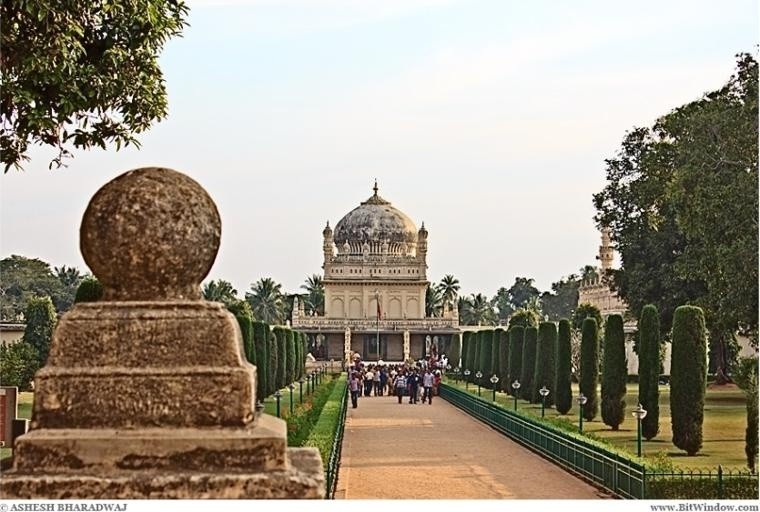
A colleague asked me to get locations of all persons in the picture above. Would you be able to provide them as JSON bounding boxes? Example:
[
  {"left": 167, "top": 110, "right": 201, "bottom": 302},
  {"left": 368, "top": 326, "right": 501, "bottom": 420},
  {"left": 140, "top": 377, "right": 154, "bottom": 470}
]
[{"left": 344, "top": 352, "right": 452, "bottom": 411}]
